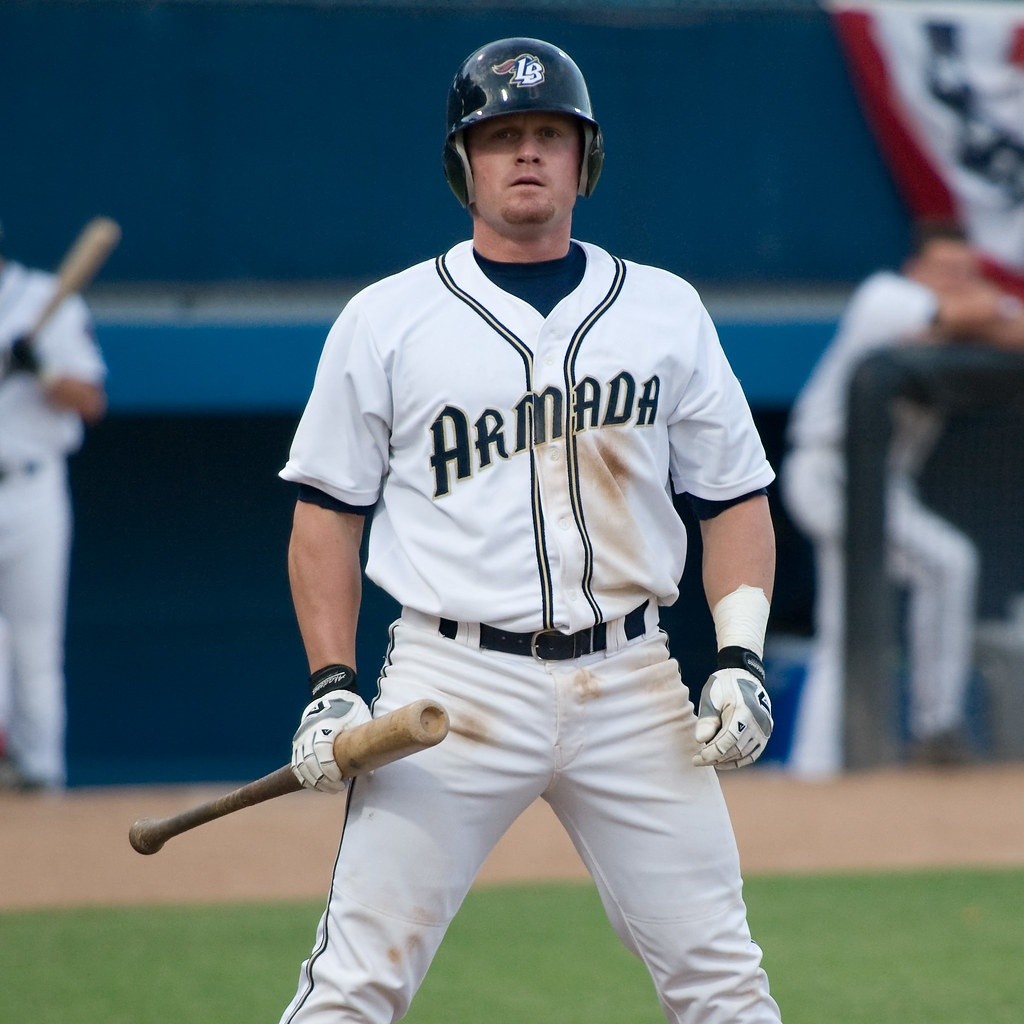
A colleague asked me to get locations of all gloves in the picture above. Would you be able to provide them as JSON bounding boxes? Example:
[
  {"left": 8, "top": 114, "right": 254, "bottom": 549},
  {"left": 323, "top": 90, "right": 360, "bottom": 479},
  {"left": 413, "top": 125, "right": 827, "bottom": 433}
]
[
  {"left": 290, "top": 662, "right": 374, "bottom": 794},
  {"left": 692, "top": 645, "right": 774, "bottom": 771}
]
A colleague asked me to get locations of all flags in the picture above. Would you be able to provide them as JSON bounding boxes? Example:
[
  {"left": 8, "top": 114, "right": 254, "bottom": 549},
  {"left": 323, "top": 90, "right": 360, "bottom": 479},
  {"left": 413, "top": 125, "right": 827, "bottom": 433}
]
[{"left": 825, "top": 0, "right": 1024, "bottom": 309}]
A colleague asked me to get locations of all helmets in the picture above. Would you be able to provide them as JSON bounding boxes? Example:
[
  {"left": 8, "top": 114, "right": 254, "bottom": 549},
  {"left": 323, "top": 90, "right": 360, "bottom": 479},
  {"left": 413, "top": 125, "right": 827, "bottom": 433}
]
[{"left": 444, "top": 37, "right": 604, "bottom": 209}]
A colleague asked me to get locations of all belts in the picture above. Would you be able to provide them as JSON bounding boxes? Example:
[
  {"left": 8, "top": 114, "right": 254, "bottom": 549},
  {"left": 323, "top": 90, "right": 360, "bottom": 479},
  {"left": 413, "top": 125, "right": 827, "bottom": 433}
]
[{"left": 438, "top": 600, "right": 649, "bottom": 663}]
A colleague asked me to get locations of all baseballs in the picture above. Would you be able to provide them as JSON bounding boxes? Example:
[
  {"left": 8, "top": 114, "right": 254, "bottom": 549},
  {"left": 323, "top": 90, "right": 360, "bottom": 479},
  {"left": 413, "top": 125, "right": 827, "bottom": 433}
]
[
  {"left": 126, "top": 697, "right": 448, "bottom": 856},
  {"left": 0, "top": 215, "right": 122, "bottom": 384}
]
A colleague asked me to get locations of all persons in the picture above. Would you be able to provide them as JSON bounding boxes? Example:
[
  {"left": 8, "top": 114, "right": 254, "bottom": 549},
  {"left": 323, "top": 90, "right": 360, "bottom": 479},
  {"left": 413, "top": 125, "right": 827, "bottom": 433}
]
[
  {"left": 784, "top": 223, "right": 1024, "bottom": 777},
  {"left": 0, "top": 261, "right": 108, "bottom": 786},
  {"left": 278, "top": 38, "right": 783, "bottom": 1024}
]
